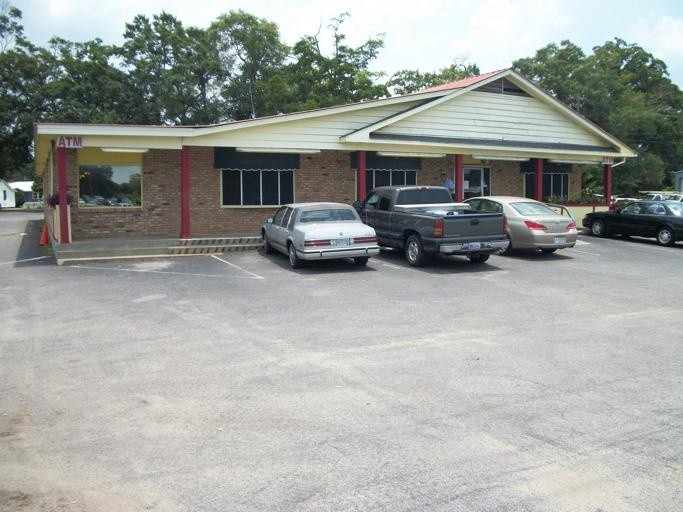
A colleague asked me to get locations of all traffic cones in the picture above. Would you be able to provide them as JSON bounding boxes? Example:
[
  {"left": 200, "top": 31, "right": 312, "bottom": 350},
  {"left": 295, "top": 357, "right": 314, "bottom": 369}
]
[{"left": 39, "top": 223, "right": 49, "bottom": 246}]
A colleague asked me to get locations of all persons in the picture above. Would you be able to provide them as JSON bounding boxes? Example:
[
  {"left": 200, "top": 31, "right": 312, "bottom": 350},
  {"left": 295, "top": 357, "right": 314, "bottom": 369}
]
[{"left": 440, "top": 171, "right": 455, "bottom": 201}]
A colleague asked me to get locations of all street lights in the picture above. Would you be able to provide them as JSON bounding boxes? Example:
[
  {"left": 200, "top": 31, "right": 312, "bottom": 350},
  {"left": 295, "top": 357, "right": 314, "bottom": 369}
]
[{"left": 245, "top": 77, "right": 258, "bottom": 119}]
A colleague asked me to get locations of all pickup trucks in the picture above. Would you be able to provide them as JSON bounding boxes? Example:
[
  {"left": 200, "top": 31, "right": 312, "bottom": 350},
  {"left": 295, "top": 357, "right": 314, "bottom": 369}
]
[{"left": 352, "top": 183, "right": 514, "bottom": 271}]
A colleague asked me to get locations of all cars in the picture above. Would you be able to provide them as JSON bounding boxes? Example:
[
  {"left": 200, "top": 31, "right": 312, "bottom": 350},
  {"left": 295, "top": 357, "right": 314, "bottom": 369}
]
[
  {"left": 580, "top": 188, "right": 683, "bottom": 248},
  {"left": 78, "top": 194, "right": 133, "bottom": 207},
  {"left": 456, "top": 192, "right": 582, "bottom": 256},
  {"left": 256, "top": 200, "right": 381, "bottom": 274}
]
